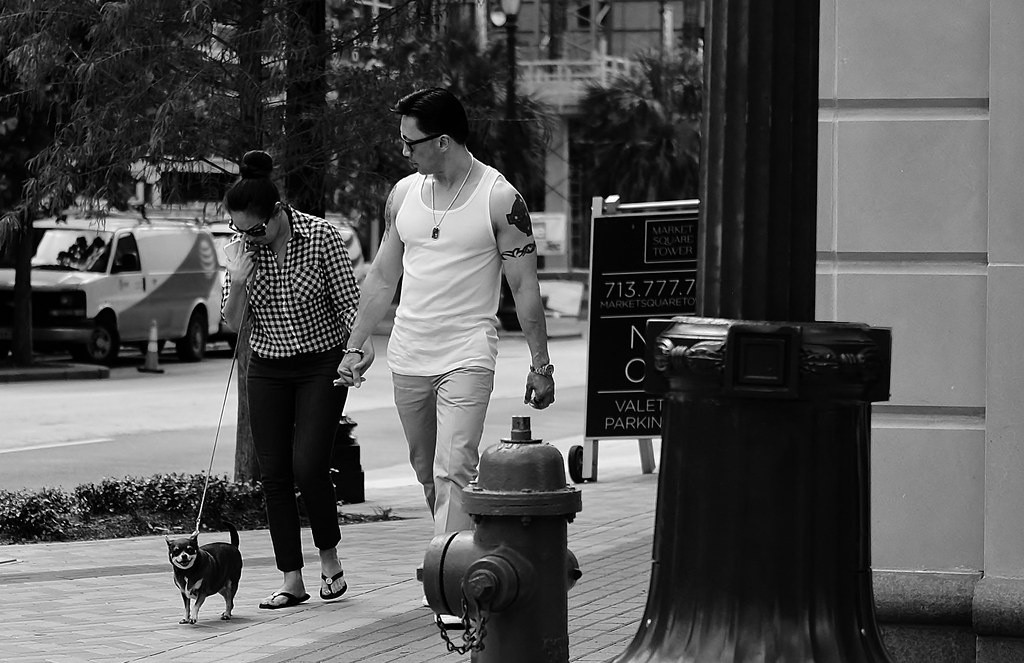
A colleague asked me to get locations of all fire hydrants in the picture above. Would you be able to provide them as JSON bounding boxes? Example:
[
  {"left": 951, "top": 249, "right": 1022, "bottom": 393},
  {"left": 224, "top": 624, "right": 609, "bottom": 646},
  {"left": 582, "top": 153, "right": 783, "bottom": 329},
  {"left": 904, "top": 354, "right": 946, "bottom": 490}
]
[{"left": 418, "top": 415, "right": 585, "bottom": 663}]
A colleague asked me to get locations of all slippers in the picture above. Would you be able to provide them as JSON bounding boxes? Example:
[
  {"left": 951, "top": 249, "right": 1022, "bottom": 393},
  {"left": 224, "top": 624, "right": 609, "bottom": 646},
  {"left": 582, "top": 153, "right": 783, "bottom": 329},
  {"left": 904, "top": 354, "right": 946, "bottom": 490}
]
[
  {"left": 319, "top": 562, "right": 347, "bottom": 600},
  {"left": 259, "top": 592, "right": 311, "bottom": 608}
]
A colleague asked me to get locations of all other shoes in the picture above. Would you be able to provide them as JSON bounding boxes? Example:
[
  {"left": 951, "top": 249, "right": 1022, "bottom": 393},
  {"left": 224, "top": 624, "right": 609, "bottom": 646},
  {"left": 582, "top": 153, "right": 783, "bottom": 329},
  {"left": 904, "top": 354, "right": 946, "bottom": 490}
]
[{"left": 432, "top": 614, "right": 473, "bottom": 628}]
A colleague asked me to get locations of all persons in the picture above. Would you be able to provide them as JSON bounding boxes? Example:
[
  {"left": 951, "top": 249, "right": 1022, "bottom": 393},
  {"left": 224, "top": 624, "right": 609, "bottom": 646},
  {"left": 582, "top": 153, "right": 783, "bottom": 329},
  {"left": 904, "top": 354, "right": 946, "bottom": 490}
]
[
  {"left": 222, "top": 149, "right": 374, "bottom": 611},
  {"left": 339, "top": 87, "right": 555, "bottom": 628}
]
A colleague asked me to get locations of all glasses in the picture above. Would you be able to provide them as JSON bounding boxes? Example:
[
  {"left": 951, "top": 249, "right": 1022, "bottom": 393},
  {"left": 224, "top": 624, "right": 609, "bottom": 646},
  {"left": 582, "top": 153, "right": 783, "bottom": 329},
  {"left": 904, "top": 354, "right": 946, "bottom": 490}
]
[
  {"left": 229, "top": 213, "right": 273, "bottom": 237},
  {"left": 398, "top": 135, "right": 441, "bottom": 152}
]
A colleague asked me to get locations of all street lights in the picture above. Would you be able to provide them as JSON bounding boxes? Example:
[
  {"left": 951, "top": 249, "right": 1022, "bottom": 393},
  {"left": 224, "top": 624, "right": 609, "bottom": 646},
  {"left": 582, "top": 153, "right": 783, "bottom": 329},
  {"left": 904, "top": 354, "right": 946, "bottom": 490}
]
[{"left": 500, "top": 0, "right": 521, "bottom": 188}]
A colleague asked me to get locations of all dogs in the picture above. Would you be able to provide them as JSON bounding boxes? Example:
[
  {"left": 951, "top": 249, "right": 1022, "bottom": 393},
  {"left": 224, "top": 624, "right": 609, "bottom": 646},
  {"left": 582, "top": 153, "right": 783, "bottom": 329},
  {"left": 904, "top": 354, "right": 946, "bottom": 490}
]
[{"left": 161, "top": 522, "right": 243, "bottom": 625}]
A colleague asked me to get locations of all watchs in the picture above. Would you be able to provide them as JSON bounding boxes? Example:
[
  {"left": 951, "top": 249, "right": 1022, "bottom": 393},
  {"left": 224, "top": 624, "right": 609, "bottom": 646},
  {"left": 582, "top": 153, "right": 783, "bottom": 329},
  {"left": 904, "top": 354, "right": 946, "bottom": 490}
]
[{"left": 529, "top": 363, "right": 554, "bottom": 377}]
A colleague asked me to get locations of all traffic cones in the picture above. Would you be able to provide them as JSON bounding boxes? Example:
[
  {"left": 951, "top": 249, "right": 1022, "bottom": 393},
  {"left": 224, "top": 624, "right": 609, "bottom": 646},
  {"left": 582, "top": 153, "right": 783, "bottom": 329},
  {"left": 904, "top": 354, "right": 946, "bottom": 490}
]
[{"left": 136, "top": 318, "right": 166, "bottom": 375}]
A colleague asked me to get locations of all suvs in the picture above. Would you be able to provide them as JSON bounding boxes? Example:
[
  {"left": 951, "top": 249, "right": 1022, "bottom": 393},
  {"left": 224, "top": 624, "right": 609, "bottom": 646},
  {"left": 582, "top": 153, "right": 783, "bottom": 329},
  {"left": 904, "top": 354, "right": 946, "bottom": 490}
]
[{"left": 0, "top": 214, "right": 367, "bottom": 367}]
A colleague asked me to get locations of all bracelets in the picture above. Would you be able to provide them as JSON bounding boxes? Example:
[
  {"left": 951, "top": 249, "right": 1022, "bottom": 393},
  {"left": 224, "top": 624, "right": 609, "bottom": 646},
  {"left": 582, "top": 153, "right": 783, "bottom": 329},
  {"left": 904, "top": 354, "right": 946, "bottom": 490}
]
[{"left": 341, "top": 347, "right": 365, "bottom": 359}]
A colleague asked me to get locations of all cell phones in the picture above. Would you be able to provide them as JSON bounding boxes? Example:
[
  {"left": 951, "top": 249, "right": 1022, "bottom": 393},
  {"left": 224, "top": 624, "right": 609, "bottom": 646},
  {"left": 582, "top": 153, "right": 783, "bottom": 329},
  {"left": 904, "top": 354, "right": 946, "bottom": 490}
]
[{"left": 223, "top": 240, "right": 241, "bottom": 262}]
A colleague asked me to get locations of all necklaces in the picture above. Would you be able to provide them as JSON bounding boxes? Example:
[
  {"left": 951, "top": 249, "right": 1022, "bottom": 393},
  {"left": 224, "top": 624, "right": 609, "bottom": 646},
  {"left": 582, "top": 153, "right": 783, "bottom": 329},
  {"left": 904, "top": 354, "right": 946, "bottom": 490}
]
[{"left": 428, "top": 154, "right": 475, "bottom": 237}]
